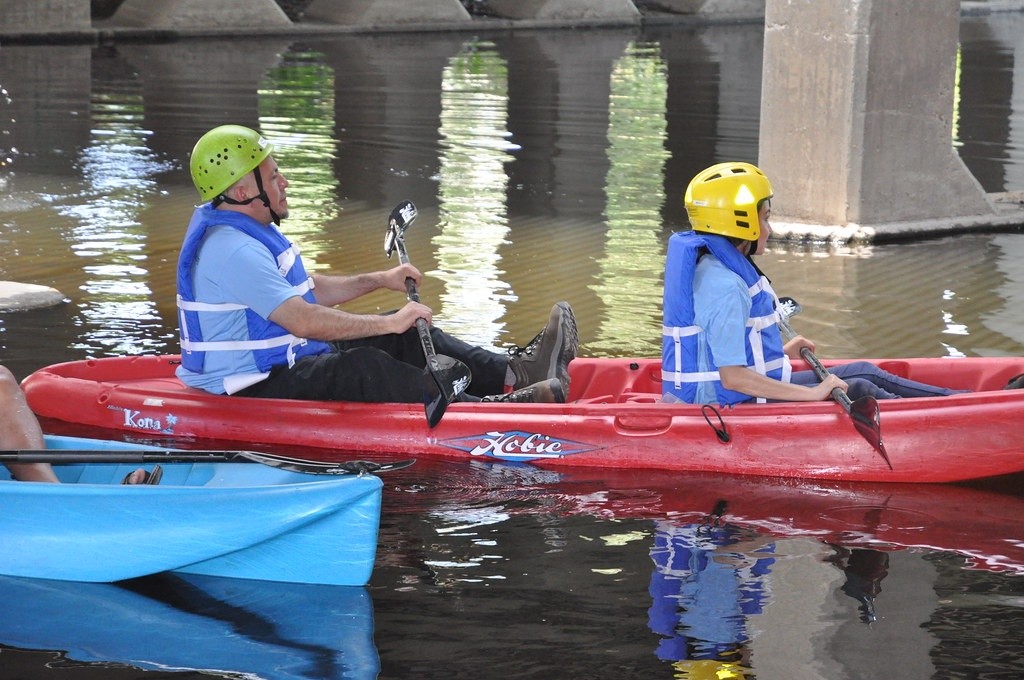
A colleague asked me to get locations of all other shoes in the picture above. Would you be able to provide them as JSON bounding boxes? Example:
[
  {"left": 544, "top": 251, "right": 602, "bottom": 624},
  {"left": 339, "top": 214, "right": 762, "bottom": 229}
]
[{"left": 120, "top": 465, "right": 163, "bottom": 485}]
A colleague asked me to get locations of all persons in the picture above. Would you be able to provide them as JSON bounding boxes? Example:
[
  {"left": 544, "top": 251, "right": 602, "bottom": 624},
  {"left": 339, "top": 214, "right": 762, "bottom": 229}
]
[
  {"left": 685, "top": 168, "right": 1023, "bottom": 407},
  {"left": 175, "top": 124, "right": 579, "bottom": 404},
  {"left": 1, "top": 362, "right": 164, "bottom": 487}
]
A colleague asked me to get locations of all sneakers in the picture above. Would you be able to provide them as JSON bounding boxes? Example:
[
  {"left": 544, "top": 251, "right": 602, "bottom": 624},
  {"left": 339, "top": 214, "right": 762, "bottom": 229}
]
[{"left": 479, "top": 299, "right": 577, "bottom": 403}]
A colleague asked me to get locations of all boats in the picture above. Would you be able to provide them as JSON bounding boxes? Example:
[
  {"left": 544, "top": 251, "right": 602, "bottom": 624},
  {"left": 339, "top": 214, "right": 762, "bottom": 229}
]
[
  {"left": 364, "top": 448, "right": 1024, "bottom": 581},
  {"left": 0, "top": 433, "right": 387, "bottom": 589},
  {"left": 20, "top": 349, "right": 1024, "bottom": 485},
  {"left": 1, "top": 572, "right": 382, "bottom": 678}
]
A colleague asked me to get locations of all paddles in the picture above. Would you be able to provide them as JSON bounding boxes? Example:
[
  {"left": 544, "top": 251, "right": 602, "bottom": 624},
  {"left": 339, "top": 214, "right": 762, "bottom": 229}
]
[
  {"left": 0, "top": 450, "right": 417, "bottom": 475},
  {"left": 383, "top": 197, "right": 473, "bottom": 429},
  {"left": 772, "top": 297, "right": 895, "bottom": 472}
]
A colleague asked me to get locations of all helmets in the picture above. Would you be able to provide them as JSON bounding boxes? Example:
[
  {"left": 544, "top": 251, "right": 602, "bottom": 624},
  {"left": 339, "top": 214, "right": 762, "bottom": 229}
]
[
  {"left": 684, "top": 162, "right": 772, "bottom": 240},
  {"left": 191, "top": 124, "right": 274, "bottom": 202}
]
